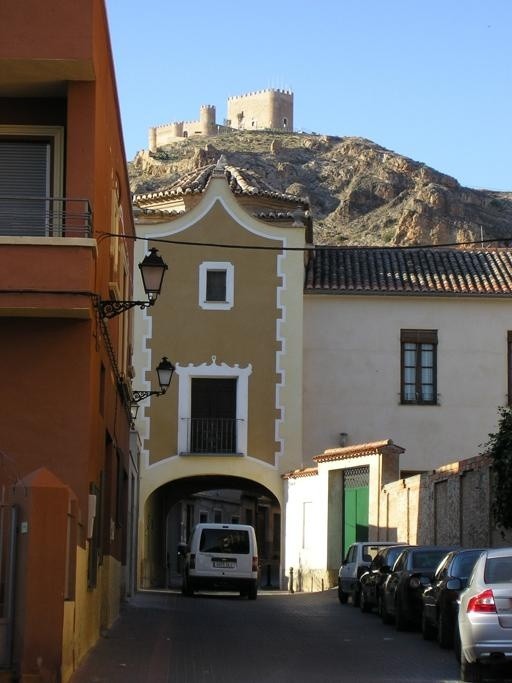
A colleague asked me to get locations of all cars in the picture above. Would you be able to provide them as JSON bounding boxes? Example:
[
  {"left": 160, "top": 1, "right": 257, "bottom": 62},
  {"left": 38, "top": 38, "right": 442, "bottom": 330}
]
[{"left": 333, "top": 538, "right": 512, "bottom": 682}]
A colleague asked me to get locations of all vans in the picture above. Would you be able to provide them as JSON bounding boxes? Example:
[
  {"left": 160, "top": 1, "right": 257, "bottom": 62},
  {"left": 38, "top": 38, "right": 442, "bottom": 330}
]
[{"left": 175, "top": 522, "right": 260, "bottom": 598}]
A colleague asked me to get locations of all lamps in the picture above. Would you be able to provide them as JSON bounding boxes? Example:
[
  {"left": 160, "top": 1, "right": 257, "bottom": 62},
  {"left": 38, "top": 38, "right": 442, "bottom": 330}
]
[
  {"left": 131, "top": 357, "right": 176, "bottom": 402},
  {"left": 98, "top": 246, "right": 169, "bottom": 319}
]
